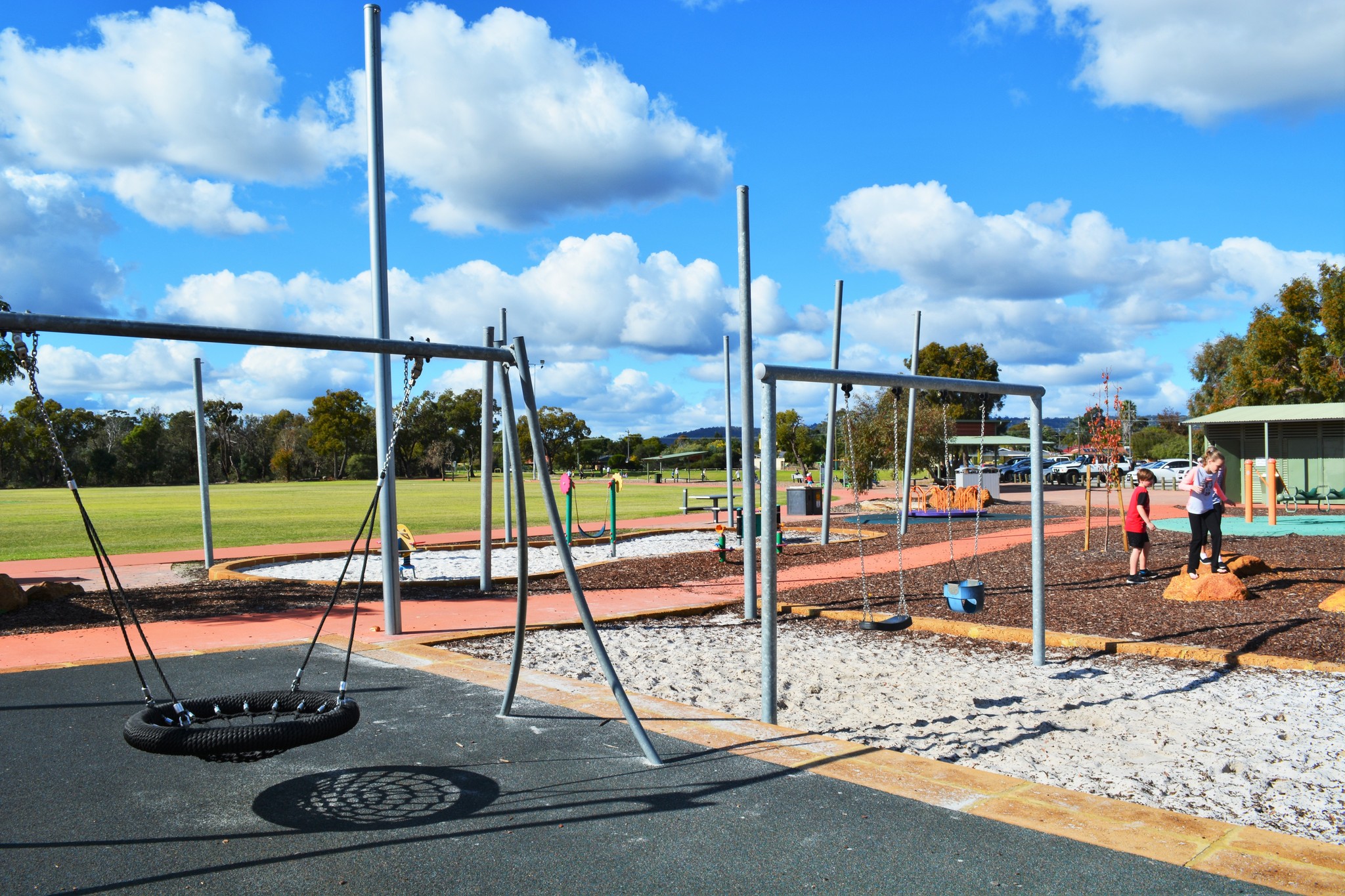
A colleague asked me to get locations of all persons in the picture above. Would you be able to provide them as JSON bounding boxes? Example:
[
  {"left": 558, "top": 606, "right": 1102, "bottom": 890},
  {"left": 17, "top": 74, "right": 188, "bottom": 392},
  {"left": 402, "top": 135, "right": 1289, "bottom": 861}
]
[
  {"left": 1124, "top": 469, "right": 1158, "bottom": 584},
  {"left": 1178, "top": 446, "right": 1237, "bottom": 580},
  {"left": 567, "top": 467, "right": 838, "bottom": 488}
]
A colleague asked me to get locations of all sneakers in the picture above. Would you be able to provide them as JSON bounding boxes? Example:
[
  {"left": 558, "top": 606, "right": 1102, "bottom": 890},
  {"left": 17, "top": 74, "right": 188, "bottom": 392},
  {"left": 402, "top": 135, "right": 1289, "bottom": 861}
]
[
  {"left": 1139, "top": 570, "right": 1158, "bottom": 578},
  {"left": 1126, "top": 574, "right": 1149, "bottom": 584}
]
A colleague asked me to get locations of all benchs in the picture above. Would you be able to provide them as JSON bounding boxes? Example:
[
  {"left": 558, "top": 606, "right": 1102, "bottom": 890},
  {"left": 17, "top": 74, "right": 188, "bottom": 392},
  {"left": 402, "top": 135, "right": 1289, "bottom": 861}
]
[
  {"left": 899, "top": 485, "right": 928, "bottom": 487},
  {"left": 679, "top": 506, "right": 713, "bottom": 509},
  {"left": 712, "top": 507, "right": 743, "bottom": 510},
  {"left": 929, "top": 483, "right": 956, "bottom": 487},
  {"left": 791, "top": 474, "right": 804, "bottom": 483}
]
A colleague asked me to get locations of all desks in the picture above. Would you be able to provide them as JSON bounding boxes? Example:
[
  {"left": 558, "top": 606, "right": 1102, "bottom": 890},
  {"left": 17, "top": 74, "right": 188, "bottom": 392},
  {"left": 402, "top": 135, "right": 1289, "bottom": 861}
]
[
  {"left": 944, "top": 479, "right": 956, "bottom": 492},
  {"left": 688, "top": 495, "right": 742, "bottom": 523},
  {"left": 911, "top": 479, "right": 923, "bottom": 492}
]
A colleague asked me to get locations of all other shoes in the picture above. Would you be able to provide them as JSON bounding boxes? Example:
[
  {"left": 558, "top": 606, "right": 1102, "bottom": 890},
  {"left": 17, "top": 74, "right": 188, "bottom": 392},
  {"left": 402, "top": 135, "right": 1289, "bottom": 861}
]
[
  {"left": 1199, "top": 555, "right": 1211, "bottom": 565},
  {"left": 1218, "top": 562, "right": 1226, "bottom": 569}
]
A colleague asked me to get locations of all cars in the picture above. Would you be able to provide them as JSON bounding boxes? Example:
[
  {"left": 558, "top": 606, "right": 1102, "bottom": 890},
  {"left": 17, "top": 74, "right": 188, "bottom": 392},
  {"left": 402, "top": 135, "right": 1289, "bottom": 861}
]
[
  {"left": 948, "top": 458, "right": 979, "bottom": 474},
  {"left": 1125, "top": 452, "right": 1204, "bottom": 487},
  {"left": 980, "top": 455, "right": 1074, "bottom": 483}
]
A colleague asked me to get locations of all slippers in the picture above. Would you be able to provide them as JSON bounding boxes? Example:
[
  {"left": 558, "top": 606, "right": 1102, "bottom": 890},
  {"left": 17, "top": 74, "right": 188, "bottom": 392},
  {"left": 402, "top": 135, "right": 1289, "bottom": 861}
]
[
  {"left": 1189, "top": 571, "right": 1199, "bottom": 580},
  {"left": 1212, "top": 567, "right": 1228, "bottom": 574}
]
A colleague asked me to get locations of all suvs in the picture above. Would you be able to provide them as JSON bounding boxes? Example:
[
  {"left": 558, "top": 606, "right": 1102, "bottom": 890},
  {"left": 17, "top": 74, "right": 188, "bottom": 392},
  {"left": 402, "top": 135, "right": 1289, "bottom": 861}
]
[{"left": 1051, "top": 453, "right": 1133, "bottom": 484}]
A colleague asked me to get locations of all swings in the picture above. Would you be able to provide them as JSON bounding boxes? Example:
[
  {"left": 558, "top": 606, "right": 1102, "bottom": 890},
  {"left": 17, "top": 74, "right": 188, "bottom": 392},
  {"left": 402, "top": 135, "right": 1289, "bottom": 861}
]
[
  {"left": 14, "top": 347, "right": 432, "bottom": 765},
  {"left": 574, "top": 481, "right": 610, "bottom": 539},
  {"left": 844, "top": 385, "right": 913, "bottom": 633},
  {"left": 938, "top": 390, "right": 987, "bottom": 614}
]
